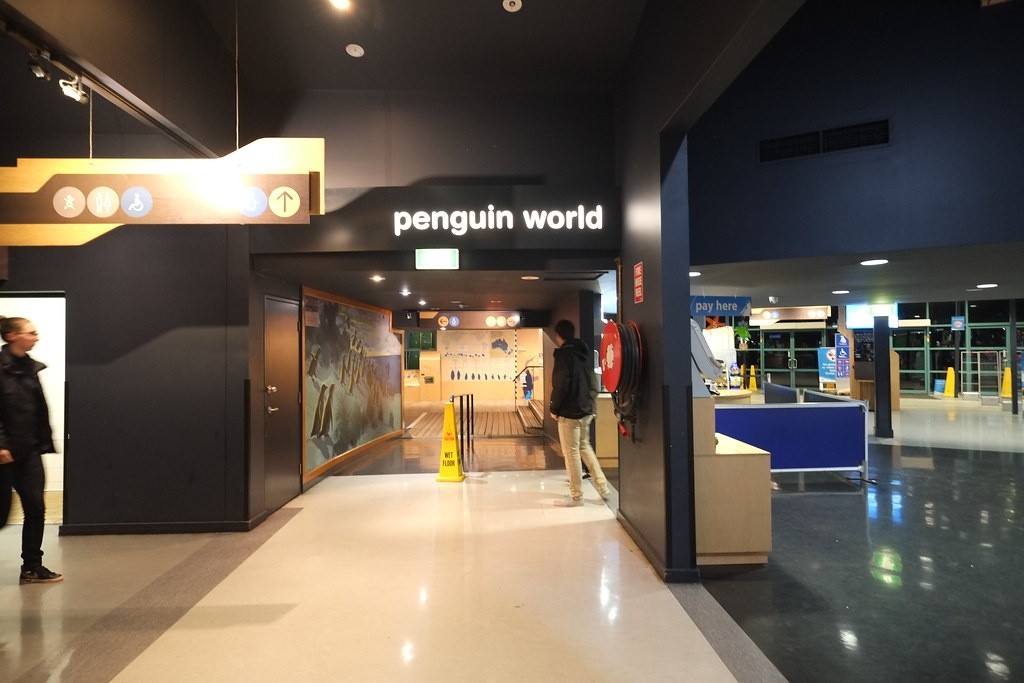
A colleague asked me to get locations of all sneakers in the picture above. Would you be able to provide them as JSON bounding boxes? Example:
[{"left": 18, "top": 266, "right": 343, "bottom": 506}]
[
  {"left": 601, "top": 488, "right": 612, "bottom": 499},
  {"left": 19, "top": 565, "right": 64, "bottom": 584},
  {"left": 553, "top": 496, "right": 584, "bottom": 506}
]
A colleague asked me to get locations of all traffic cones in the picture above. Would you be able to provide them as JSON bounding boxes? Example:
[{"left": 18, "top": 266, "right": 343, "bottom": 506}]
[
  {"left": 748, "top": 365, "right": 758, "bottom": 391},
  {"left": 999, "top": 368, "right": 1014, "bottom": 397},
  {"left": 942, "top": 366, "right": 956, "bottom": 396},
  {"left": 739, "top": 365, "right": 747, "bottom": 389},
  {"left": 437, "top": 402, "right": 467, "bottom": 483}
]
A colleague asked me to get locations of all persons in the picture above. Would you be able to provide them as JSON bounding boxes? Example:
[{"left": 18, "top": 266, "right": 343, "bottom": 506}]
[
  {"left": 550, "top": 319, "right": 610, "bottom": 506},
  {"left": 523, "top": 370, "right": 533, "bottom": 399},
  {"left": 0, "top": 314, "right": 63, "bottom": 583}
]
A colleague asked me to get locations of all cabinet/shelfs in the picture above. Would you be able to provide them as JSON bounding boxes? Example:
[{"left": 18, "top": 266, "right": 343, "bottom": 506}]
[
  {"left": 596, "top": 394, "right": 619, "bottom": 468},
  {"left": 693, "top": 398, "right": 773, "bottom": 566},
  {"left": 849, "top": 329, "right": 874, "bottom": 410}
]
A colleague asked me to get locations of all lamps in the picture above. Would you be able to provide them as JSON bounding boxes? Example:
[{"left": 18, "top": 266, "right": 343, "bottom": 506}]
[
  {"left": 29, "top": 47, "right": 51, "bottom": 79},
  {"left": 59, "top": 72, "right": 89, "bottom": 105}
]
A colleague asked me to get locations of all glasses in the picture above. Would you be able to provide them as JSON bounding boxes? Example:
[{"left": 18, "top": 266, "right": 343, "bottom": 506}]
[{"left": 16, "top": 332, "right": 38, "bottom": 336}]
